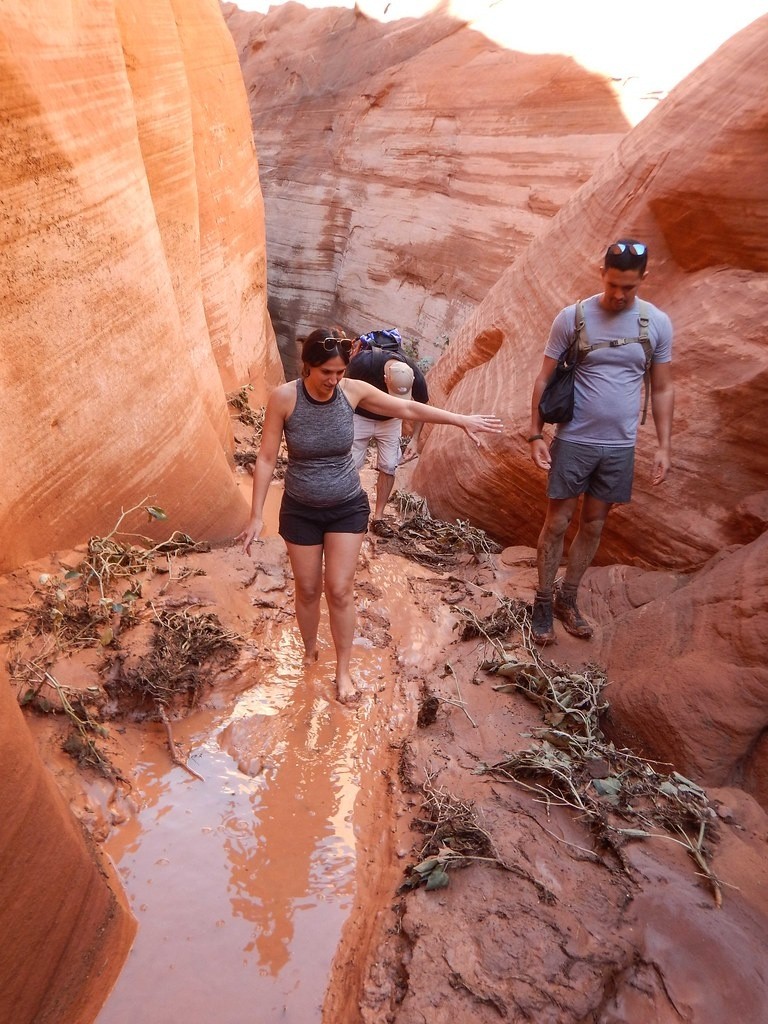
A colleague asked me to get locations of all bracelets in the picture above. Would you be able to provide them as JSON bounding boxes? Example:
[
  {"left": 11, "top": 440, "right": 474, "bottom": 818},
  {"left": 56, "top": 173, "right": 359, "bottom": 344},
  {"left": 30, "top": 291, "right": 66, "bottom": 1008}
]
[{"left": 528, "top": 435, "right": 543, "bottom": 443}]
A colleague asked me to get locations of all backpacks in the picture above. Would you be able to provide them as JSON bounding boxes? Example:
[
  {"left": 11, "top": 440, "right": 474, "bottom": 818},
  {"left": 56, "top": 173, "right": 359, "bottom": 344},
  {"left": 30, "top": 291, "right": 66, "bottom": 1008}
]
[{"left": 347, "top": 327, "right": 407, "bottom": 385}]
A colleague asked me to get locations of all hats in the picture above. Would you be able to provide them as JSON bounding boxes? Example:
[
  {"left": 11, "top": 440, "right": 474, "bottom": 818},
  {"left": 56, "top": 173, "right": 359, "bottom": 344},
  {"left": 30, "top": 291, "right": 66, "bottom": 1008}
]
[{"left": 387, "top": 362, "right": 413, "bottom": 400}]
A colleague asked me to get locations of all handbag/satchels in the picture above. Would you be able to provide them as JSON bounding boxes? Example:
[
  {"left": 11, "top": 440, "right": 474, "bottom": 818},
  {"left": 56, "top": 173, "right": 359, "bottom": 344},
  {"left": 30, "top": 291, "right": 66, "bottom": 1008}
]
[{"left": 537, "top": 299, "right": 585, "bottom": 426}]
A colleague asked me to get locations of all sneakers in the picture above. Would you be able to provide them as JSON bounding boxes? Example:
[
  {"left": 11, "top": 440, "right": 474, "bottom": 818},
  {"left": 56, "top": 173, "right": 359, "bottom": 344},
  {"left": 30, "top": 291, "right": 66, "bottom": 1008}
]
[
  {"left": 531, "top": 600, "right": 557, "bottom": 644},
  {"left": 552, "top": 587, "right": 593, "bottom": 637}
]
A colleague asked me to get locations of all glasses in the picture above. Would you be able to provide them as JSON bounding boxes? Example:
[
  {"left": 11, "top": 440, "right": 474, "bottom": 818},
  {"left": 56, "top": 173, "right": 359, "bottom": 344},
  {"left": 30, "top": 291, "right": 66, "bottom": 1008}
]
[
  {"left": 607, "top": 243, "right": 647, "bottom": 255},
  {"left": 315, "top": 338, "right": 353, "bottom": 352}
]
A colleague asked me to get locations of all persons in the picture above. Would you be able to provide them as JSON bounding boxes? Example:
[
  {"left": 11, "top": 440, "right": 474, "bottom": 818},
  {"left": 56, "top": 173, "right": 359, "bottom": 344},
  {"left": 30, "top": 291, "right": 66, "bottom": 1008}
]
[
  {"left": 530, "top": 239, "right": 674, "bottom": 643},
  {"left": 234, "top": 328, "right": 504, "bottom": 704},
  {"left": 340, "top": 349, "right": 430, "bottom": 537}
]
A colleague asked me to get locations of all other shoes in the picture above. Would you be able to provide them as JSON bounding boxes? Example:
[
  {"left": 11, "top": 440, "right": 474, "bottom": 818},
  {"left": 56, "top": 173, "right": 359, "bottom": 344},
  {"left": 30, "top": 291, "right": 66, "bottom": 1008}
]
[{"left": 369, "top": 519, "right": 394, "bottom": 538}]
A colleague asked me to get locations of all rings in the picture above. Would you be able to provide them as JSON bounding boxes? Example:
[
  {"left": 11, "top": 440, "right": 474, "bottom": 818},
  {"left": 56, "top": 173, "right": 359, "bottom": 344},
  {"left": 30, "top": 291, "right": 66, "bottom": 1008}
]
[{"left": 485, "top": 419, "right": 487, "bottom": 422}]
